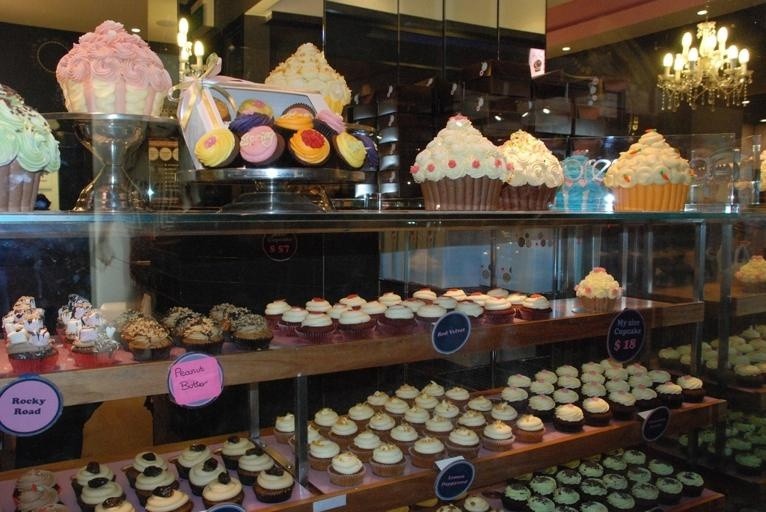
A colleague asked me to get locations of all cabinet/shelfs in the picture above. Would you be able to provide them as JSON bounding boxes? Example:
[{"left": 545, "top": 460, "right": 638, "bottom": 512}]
[
  {"left": 0, "top": 204, "right": 736, "bottom": 512},
  {"left": 345, "top": 89, "right": 436, "bottom": 205},
  {"left": 575, "top": 202, "right": 765, "bottom": 512}
]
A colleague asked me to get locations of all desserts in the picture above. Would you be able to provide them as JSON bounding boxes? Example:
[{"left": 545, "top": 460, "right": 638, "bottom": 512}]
[
  {"left": 11, "top": 320, "right": 766, "bottom": 512},
  {"left": 2, "top": 280, "right": 554, "bottom": 366}
]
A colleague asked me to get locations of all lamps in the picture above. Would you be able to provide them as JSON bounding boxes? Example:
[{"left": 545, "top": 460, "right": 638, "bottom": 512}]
[
  {"left": 173, "top": 13, "right": 207, "bottom": 87},
  {"left": 658, "top": 21, "right": 753, "bottom": 114}
]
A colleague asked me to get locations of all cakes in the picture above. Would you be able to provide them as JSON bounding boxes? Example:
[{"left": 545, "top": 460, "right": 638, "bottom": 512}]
[
  {"left": 733, "top": 254, "right": 766, "bottom": 294},
  {"left": 573, "top": 266, "right": 623, "bottom": 312},
  {"left": 492, "top": 128, "right": 564, "bottom": 212},
  {"left": 0, "top": 79, "right": 63, "bottom": 214},
  {"left": 54, "top": 18, "right": 173, "bottom": 118},
  {"left": 166, "top": 43, "right": 380, "bottom": 172},
  {"left": 407, "top": 113, "right": 509, "bottom": 211},
  {"left": 602, "top": 127, "right": 694, "bottom": 214},
  {"left": 552, "top": 155, "right": 612, "bottom": 214}
]
